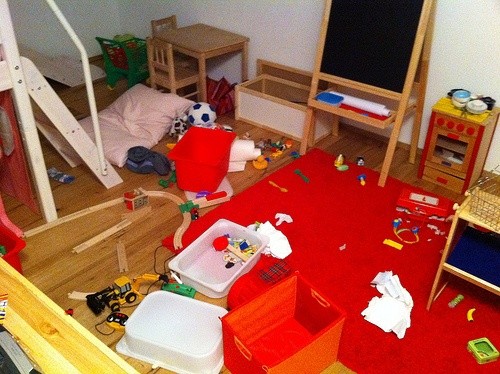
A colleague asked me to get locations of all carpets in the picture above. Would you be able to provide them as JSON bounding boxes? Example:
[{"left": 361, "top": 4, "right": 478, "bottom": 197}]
[{"left": 162, "top": 147, "right": 500, "bottom": 374}]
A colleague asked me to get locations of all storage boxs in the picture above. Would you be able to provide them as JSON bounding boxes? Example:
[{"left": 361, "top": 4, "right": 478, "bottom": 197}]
[
  {"left": 169, "top": 218, "right": 268, "bottom": 297},
  {"left": 167, "top": 124, "right": 236, "bottom": 194},
  {"left": 116, "top": 291, "right": 229, "bottom": 374},
  {"left": 219, "top": 272, "right": 347, "bottom": 374}
]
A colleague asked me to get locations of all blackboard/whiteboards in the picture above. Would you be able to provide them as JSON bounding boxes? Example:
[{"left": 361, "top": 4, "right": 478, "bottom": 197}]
[{"left": 320, "top": 0, "right": 440, "bottom": 100}]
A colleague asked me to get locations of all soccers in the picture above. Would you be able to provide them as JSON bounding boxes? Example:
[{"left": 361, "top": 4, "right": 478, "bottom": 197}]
[{"left": 188, "top": 101, "right": 217, "bottom": 125}]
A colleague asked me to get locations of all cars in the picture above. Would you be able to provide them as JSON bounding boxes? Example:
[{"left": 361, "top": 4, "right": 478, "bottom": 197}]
[{"left": 161, "top": 282, "right": 196, "bottom": 298}]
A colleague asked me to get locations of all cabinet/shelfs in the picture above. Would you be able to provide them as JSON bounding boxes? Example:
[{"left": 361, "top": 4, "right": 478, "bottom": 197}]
[
  {"left": 425, "top": 175, "right": 500, "bottom": 309},
  {"left": 417, "top": 97, "right": 499, "bottom": 195}
]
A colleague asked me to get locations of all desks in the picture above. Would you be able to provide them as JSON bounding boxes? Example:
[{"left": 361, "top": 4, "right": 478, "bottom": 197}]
[{"left": 158, "top": 23, "right": 249, "bottom": 103}]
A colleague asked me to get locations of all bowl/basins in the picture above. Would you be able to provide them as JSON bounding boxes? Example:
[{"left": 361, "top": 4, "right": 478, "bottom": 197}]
[
  {"left": 452, "top": 90, "right": 471, "bottom": 108},
  {"left": 479, "top": 97, "right": 496, "bottom": 110},
  {"left": 466, "top": 99, "right": 488, "bottom": 114}
]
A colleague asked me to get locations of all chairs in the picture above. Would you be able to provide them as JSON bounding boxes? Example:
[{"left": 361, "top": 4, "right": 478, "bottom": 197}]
[
  {"left": 147, "top": 37, "right": 198, "bottom": 100},
  {"left": 152, "top": 17, "right": 195, "bottom": 69}
]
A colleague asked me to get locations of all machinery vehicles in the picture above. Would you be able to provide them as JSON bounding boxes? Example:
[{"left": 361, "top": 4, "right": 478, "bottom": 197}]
[{"left": 85, "top": 273, "right": 169, "bottom": 316}]
